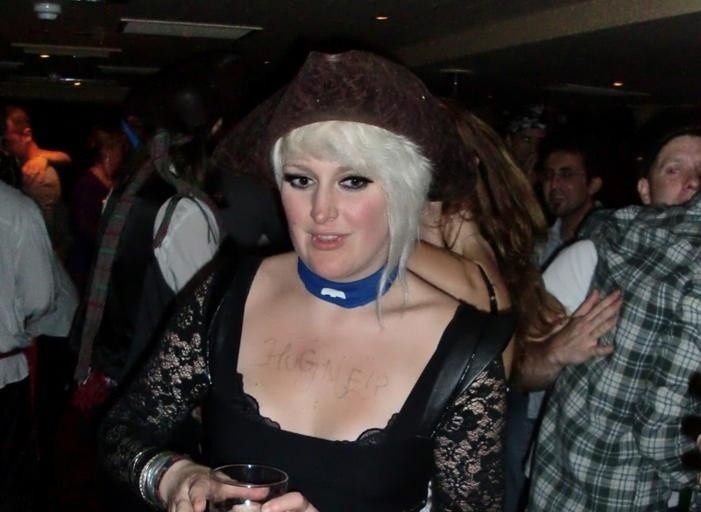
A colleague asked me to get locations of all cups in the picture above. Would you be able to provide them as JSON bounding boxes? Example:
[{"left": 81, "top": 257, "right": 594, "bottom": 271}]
[{"left": 207, "top": 463, "right": 290, "bottom": 512}]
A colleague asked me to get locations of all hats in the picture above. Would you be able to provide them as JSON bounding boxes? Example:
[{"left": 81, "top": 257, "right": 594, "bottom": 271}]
[{"left": 257, "top": 51, "right": 446, "bottom": 187}]
